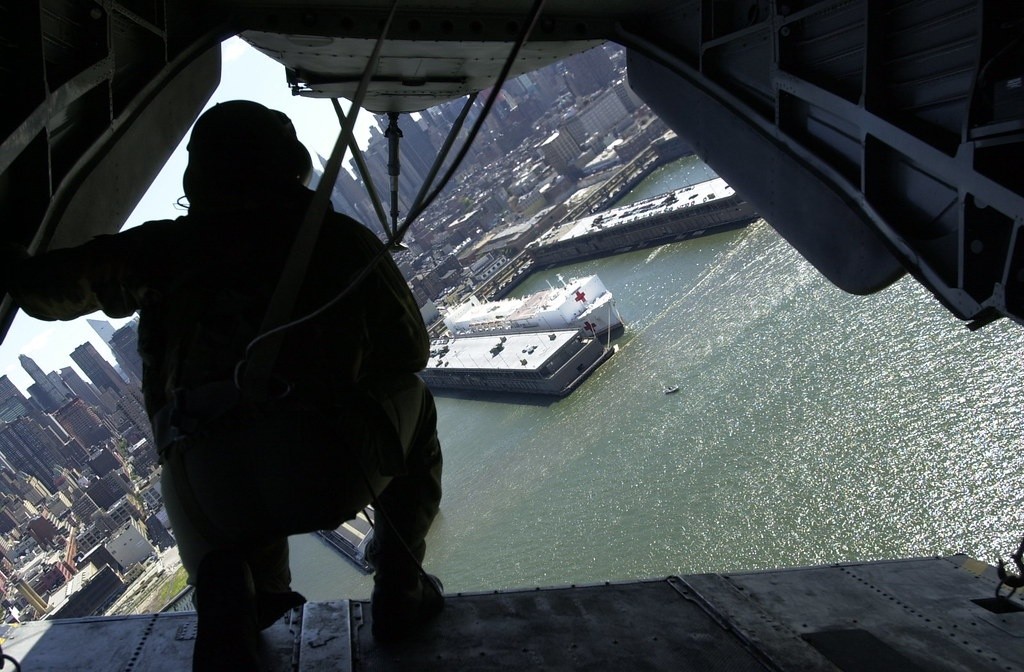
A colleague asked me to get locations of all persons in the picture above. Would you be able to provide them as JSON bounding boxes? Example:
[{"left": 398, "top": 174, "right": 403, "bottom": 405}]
[{"left": 0, "top": 100, "right": 444, "bottom": 672}]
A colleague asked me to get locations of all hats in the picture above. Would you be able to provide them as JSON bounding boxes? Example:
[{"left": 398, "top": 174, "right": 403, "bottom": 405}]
[{"left": 185, "top": 99, "right": 309, "bottom": 199}]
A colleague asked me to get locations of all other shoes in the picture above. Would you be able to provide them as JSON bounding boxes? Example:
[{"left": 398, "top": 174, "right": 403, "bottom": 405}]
[
  {"left": 192, "top": 561, "right": 254, "bottom": 672},
  {"left": 371, "top": 573, "right": 443, "bottom": 644}
]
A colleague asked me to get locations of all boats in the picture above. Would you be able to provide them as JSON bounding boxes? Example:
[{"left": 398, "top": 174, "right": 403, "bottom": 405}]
[{"left": 434, "top": 269, "right": 624, "bottom": 337}]
